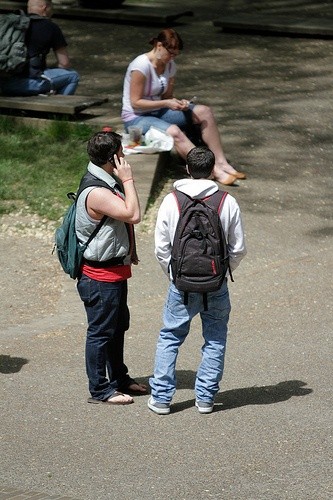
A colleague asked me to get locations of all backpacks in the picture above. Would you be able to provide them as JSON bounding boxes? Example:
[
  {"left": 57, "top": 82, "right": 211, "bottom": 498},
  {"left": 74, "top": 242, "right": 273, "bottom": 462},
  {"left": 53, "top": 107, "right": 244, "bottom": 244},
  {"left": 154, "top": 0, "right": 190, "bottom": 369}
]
[
  {"left": 0, "top": 9, "right": 51, "bottom": 78},
  {"left": 51, "top": 180, "right": 116, "bottom": 280},
  {"left": 168, "top": 189, "right": 234, "bottom": 311}
]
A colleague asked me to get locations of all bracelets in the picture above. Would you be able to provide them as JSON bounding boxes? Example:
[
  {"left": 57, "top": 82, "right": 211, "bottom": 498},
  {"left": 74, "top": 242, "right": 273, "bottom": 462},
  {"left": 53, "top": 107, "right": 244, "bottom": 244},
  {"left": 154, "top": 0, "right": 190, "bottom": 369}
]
[{"left": 122, "top": 178, "right": 135, "bottom": 183}]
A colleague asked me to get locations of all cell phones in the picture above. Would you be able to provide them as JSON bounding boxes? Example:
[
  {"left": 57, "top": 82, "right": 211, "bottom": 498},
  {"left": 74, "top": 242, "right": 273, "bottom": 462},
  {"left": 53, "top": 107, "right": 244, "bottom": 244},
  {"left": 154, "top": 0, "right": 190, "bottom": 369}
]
[
  {"left": 109, "top": 155, "right": 120, "bottom": 168},
  {"left": 188, "top": 96, "right": 196, "bottom": 106}
]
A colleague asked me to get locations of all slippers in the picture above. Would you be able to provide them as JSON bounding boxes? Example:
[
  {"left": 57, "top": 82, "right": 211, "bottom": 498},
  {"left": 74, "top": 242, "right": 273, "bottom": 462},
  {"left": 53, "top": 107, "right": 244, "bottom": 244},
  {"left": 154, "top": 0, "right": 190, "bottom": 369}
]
[
  {"left": 115, "top": 382, "right": 148, "bottom": 395},
  {"left": 86, "top": 391, "right": 133, "bottom": 405}
]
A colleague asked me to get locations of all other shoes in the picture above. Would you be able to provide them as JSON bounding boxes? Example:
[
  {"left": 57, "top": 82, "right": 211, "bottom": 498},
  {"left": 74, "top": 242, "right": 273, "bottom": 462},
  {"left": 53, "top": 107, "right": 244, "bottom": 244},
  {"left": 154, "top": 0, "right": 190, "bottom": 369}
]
[
  {"left": 216, "top": 176, "right": 236, "bottom": 185},
  {"left": 231, "top": 173, "right": 245, "bottom": 179}
]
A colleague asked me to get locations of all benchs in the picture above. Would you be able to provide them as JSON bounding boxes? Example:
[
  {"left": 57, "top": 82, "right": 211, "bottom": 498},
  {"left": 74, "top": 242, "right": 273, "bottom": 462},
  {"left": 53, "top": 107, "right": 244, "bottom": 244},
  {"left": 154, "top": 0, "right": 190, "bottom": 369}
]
[
  {"left": 0, "top": 2, "right": 27, "bottom": 10},
  {"left": 0, "top": 93, "right": 108, "bottom": 115},
  {"left": 214, "top": 15, "right": 333, "bottom": 36},
  {"left": 51, "top": 6, "right": 194, "bottom": 24}
]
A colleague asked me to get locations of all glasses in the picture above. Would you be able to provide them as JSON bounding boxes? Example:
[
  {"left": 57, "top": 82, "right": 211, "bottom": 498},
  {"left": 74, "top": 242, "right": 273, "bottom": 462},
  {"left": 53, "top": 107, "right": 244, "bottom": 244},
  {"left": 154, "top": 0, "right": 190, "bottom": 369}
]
[{"left": 164, "top": 47, "right": 178, "bottom": 57}]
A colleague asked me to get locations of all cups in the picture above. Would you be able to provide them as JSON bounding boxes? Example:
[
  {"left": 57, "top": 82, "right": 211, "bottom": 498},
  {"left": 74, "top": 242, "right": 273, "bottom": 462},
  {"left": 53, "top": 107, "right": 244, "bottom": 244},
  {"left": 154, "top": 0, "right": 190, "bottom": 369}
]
[{"left": 127, "top": 125, "right": 143, "bottom": 145}]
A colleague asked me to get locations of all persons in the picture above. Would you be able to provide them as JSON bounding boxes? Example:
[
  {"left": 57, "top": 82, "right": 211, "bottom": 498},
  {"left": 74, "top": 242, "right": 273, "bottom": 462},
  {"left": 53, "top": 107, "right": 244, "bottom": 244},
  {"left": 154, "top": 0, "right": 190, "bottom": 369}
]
[
  {"left": 0, "top": 0, "right": 81, "bottom": 96},
  {"left": 147, "top": 146, "right": 247, "bottom": 415},
  {"left": 121, "top": 30, "right": 246, "bottom": 185},
  {"left": 74, "top": 131, "right": 151, "bottom": 406}
]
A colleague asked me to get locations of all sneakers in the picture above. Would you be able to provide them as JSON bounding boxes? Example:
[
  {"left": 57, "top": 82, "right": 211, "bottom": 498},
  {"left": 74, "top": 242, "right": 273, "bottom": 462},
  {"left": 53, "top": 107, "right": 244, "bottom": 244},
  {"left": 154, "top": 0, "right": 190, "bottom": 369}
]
[
  {"left": 148, "top": 396, "right": 170, "bottom": 414},
  {"left": 196, "top": 402, "right": 214, "bottom": 413}
]
